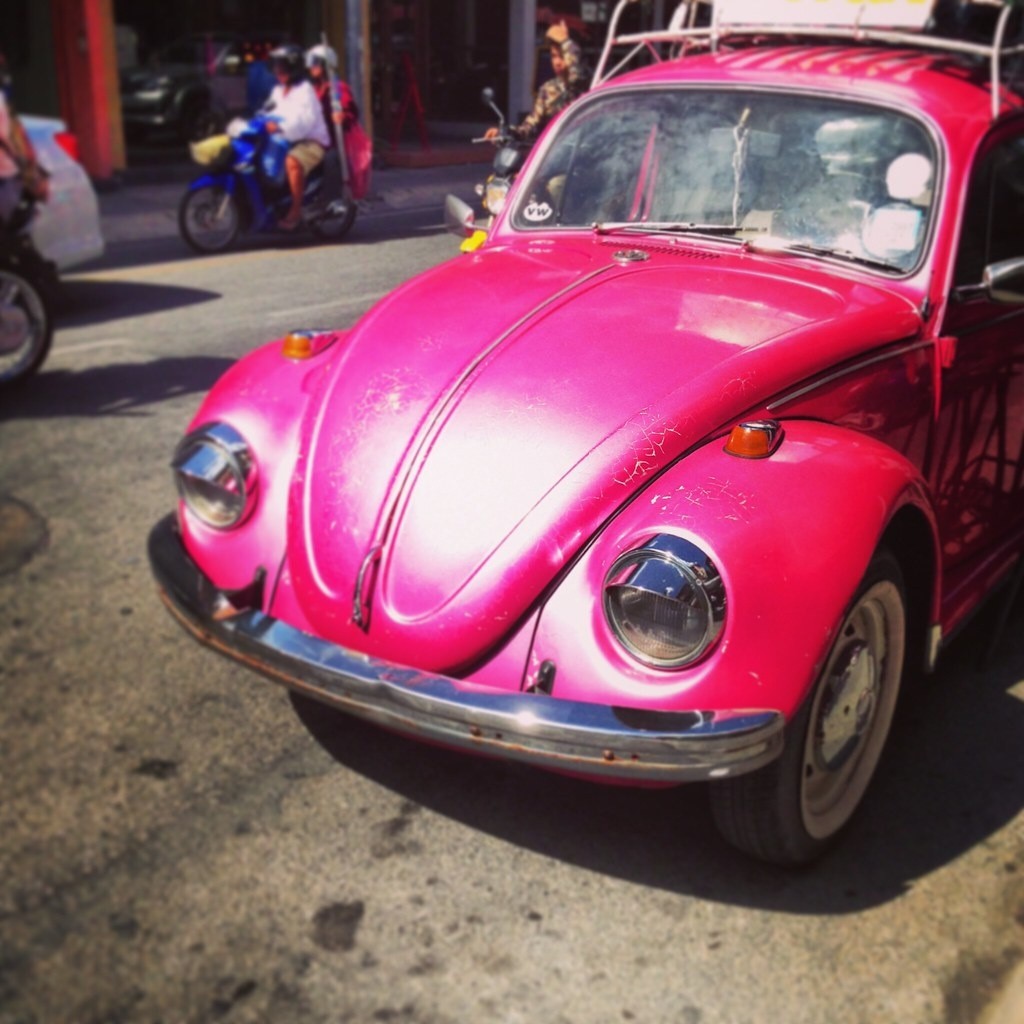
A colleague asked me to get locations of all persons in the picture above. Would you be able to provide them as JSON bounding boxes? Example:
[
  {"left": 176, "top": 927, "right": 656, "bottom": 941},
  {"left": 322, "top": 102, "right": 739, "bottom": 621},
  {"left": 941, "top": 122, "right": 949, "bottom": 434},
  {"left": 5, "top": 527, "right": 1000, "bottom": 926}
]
[
  {"left": 0, "top": 86, "right": 51, "bottom": 235},
  {"left": 241, "top": 35, "right": 370, "bottom": 232},
  {"left": 485, "top": 11, "right": 604, "bottom": 173}
]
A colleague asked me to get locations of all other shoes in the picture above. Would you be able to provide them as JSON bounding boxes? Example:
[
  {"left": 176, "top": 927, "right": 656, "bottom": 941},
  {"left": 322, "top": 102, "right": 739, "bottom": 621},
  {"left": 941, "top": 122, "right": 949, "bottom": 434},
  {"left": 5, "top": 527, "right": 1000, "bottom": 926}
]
[{"left": 278, "top": 216, "right": 303, "bottom": 231}]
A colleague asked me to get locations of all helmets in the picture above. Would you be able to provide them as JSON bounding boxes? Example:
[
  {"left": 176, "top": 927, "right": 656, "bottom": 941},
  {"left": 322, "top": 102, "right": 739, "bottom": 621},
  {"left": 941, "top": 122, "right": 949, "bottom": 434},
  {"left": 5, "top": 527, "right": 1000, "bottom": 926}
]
[
  {"left": 265, "top": 44, "right": 305, "bottom": 85},
  {"left": 302, "top": 45, "right": 338, "bottom": 74}
]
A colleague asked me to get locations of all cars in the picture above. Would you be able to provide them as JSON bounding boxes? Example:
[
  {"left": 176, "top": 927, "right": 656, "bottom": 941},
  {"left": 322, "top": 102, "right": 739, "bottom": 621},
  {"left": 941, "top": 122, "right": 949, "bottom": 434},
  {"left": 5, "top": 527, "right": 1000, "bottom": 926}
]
[
  {"left": 141, "top": 2, "right": 1024, "bottom": 881},
  {"left": 20, "top": 113, "right": 108, "bottom": 273},
  {"left": 122, "top": 33, "right": 277, "bottom": 151}
]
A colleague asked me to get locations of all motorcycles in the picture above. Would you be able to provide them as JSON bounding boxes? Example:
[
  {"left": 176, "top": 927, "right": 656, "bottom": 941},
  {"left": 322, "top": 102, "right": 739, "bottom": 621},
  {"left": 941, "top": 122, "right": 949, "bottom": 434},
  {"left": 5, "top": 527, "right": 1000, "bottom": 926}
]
[
  {"left": 174, "top": 105, "right": 360, "bottom": 252},
  {"left": 0, "top": 181, "right": 56, "bottom": 387},
  {"left": 466, "top": 85, "right": 550, "bottom": 230}
]
